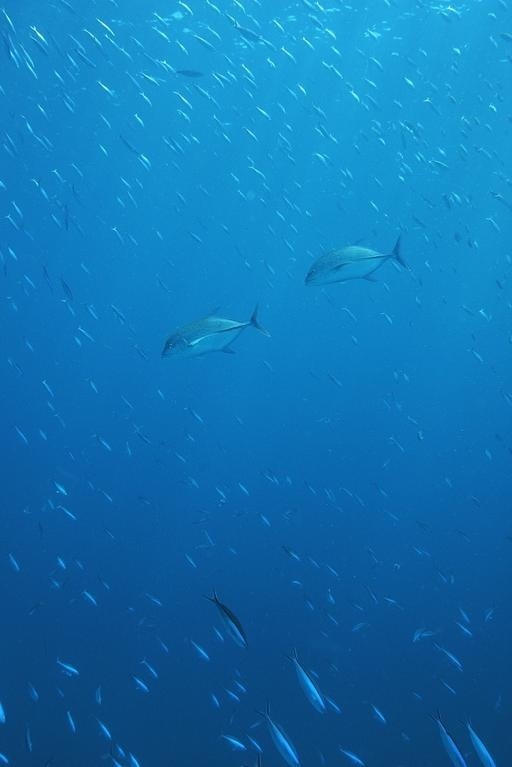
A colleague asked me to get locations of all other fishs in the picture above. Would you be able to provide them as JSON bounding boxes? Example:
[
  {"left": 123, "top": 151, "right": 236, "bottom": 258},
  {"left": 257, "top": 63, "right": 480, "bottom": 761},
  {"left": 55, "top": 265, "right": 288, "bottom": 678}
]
[{"left": 0, "top": 1, "right": 511, "bottom": 767}]
